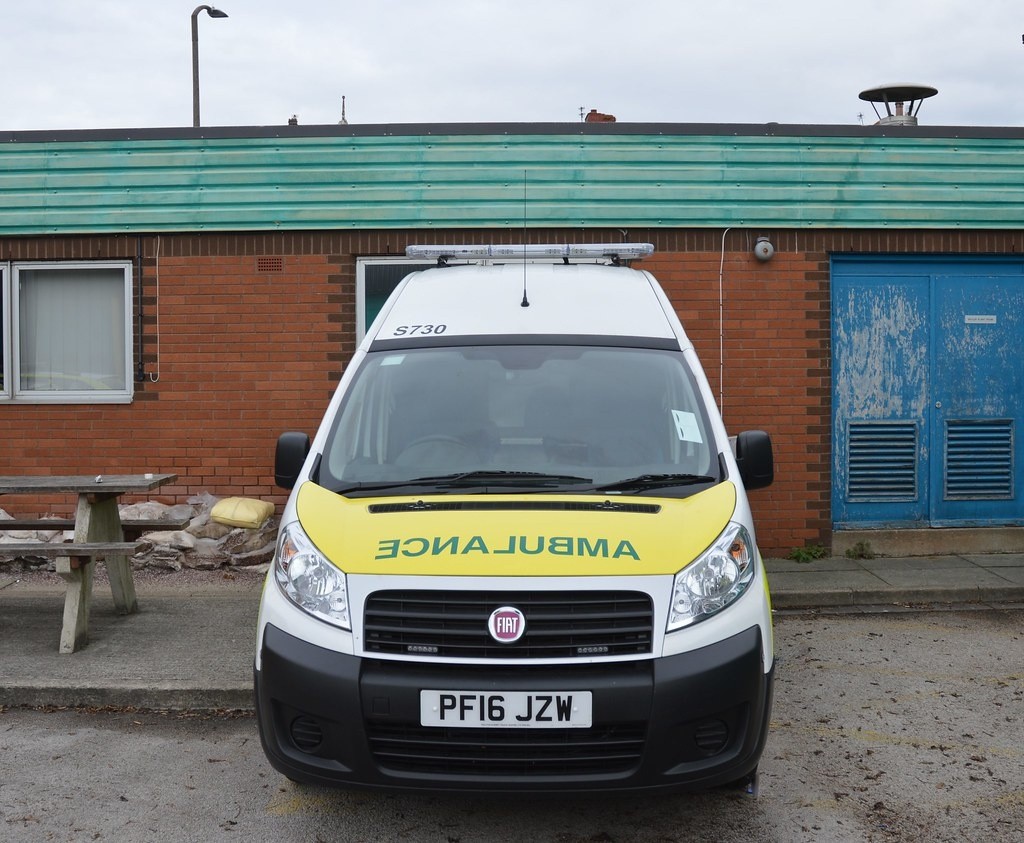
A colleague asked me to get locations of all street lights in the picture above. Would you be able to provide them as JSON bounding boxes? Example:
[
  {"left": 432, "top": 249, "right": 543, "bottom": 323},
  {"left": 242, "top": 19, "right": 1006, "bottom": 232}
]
[{"left": 189, "top": 4, "right": 233, "bottom": 129}]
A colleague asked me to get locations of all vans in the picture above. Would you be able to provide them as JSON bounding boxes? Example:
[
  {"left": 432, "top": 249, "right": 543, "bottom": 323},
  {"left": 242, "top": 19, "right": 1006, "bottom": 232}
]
[{"left": 254, "top": 242, "right": 779, "bottom": 794}]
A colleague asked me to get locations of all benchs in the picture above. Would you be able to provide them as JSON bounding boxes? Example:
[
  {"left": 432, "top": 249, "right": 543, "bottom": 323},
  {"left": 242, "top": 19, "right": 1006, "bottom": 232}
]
[
  {"left": 0, "top": 542, "right": 151, "bottom": 557},
  {"left": 0, "top": 519, "right": 190, "bottom": 531}
]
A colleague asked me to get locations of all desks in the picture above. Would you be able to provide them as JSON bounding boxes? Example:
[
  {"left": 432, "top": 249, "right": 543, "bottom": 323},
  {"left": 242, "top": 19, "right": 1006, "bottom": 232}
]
[{"left": 0, "top": 473, "right": 179, "bottom": 654}]
